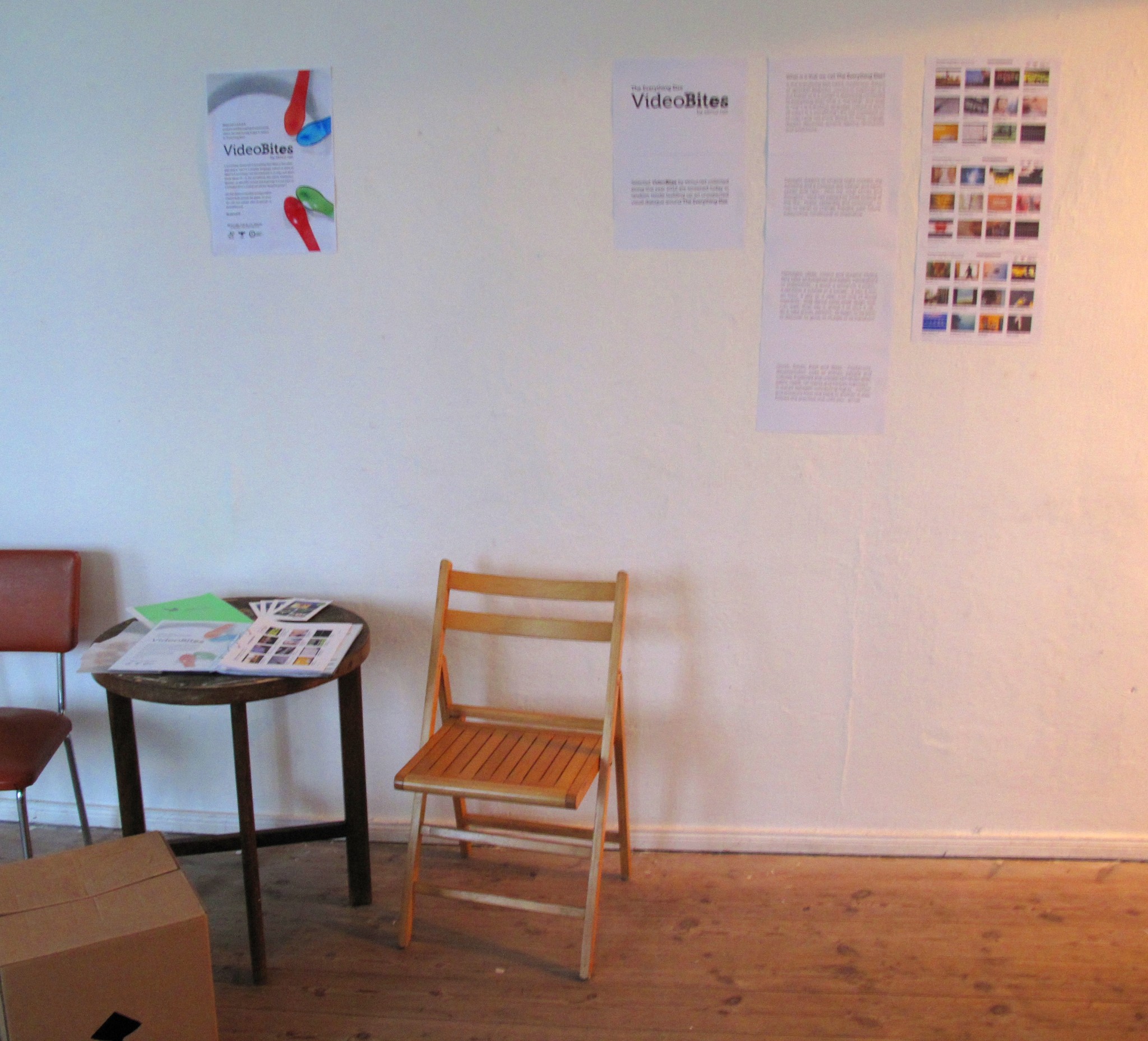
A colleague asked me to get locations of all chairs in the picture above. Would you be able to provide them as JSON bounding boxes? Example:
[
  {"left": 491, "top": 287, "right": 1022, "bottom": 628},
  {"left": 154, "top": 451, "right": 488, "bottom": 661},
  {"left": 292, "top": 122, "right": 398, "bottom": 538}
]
[
  {"left": 393, "top": 559, "right": 632, "bottom": 980},
  {"left": 0, "top": 549, "right": 92, "bottom": 860}
]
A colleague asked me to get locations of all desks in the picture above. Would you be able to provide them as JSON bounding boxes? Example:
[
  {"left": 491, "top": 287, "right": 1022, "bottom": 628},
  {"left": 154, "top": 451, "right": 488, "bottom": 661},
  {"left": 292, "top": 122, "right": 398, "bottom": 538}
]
[{"left": 91, "top": 597, "right": 371, "bottom": 985}]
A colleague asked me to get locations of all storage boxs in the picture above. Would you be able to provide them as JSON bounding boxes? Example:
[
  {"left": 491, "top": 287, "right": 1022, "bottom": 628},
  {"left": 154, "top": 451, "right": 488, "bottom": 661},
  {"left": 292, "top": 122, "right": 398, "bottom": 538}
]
[{"left": 0, "top": 830, "right": 219, "bottom": 1041}]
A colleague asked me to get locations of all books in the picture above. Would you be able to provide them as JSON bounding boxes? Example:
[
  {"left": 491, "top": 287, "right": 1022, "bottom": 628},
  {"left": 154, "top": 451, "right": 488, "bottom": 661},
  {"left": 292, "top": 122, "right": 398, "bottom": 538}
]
[
  {"left": 109, "top": 618, "right": 361, "bottom": 676},
  {"left": 125, "top": 594, "right": 255, "bottom": 629}
]
